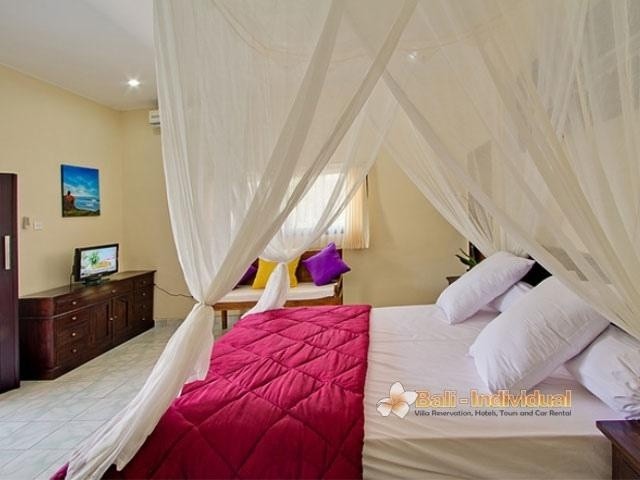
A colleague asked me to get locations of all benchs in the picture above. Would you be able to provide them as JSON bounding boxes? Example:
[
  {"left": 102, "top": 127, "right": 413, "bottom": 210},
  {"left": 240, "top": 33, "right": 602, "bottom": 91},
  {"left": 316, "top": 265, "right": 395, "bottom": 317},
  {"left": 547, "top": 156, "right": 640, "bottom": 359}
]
[{"left": 212, "top": 248, "right": 351, "bottom": 330}]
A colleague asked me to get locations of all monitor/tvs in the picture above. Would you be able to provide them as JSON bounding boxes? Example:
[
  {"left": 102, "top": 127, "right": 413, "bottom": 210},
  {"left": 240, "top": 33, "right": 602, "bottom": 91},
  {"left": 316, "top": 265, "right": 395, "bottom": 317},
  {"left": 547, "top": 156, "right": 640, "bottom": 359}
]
[{"left": 74, "top": 243, "right": 118, "bottom": 285}]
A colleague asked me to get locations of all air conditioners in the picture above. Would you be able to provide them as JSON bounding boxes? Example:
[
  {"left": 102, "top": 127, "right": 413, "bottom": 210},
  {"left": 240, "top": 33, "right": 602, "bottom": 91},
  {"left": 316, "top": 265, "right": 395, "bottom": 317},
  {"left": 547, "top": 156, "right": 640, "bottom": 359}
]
[{"left": 149, "top": 110, "right": 161, "bottom": 127}]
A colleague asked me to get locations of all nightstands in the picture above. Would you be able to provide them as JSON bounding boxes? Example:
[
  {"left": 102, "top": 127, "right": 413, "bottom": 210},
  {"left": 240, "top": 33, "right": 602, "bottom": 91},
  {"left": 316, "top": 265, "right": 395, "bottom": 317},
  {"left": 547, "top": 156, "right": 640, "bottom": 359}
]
[{"left": 593, "top": 418, "right": 639, "bottom": 480}]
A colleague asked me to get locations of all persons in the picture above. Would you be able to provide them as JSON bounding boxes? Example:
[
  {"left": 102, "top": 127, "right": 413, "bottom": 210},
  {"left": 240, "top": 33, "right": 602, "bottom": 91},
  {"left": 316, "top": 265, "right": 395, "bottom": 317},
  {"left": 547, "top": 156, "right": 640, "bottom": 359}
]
[{"left": 64, "top": 190, "right": 75, "bottom": 205}]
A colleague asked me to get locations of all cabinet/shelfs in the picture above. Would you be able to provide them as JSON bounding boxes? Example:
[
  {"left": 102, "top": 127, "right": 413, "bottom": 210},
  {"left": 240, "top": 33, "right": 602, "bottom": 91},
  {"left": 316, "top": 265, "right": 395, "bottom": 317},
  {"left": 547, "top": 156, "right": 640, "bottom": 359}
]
[
  {"left": 0, "top": 172, "right": 21, "bottom": 395},
  {"left": 20, "top": 268, "right": 158, "bottom": 381}
]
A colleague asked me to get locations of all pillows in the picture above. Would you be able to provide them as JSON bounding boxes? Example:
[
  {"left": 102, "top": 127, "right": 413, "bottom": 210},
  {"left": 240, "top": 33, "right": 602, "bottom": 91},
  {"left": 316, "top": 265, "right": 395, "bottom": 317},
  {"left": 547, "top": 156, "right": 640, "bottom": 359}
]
[
  {"left": 485, "top": 282, "right": 534, "bottom": 311},
  {"left": 435, "top": 251, "right": 536, "bottom": 325},
  {"left": 253, "top": 253, "right": 300, "bottom": 287},
  {"left": 472, "top": 275, "right": 627, "bottom": 402},
  {"left": 563, "top": 324, "right": 639, "bottom": 415},
  {"left": 303, "top": 241, "right": 351, "bottom": 286},
  {"left": 238, "top": 264, "right": 257, "bottom": 282}
]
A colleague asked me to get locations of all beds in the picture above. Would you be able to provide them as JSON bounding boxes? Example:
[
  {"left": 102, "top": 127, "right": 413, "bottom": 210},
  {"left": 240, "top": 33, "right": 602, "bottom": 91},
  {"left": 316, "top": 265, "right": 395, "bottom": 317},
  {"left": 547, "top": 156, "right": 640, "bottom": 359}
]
[{"left": 48, "top": 243, "right": 640, "bottom": 477}]
